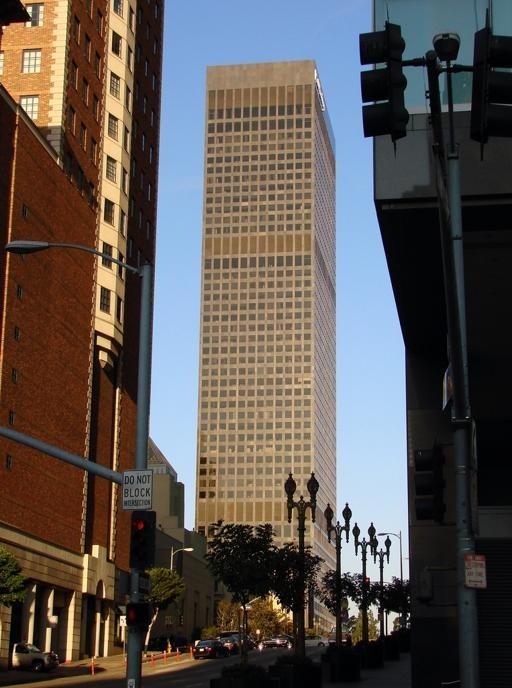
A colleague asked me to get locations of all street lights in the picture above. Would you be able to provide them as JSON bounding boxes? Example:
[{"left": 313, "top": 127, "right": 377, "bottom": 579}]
[
  {"left": 324, "top": 502, "right": 351, "bottom": 647},
  {"left": 171, "top": 545, "right": 194, "bottom": 575},
  {"left": 284, "top": 470, "right": 319, "bottom": 660},
  {"left": 241, "top": 604, "right": 252, "bottom": 631},
  {"left": 370, "top": 535, "right": 391, "bottom": 637},
  {"left": 379, "top": 531, "right": 404, "bottom": 590},
  {"left": 352, "top": 520, "right": 376, "bottom": 642},
  {"left": 4, "top": 238, "right": 154, "bottom": 688}
]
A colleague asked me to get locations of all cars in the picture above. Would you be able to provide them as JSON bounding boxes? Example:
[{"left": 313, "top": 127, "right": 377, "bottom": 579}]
[
  {"left": 304, "top": 634, "right": 329, "bottom": 647},
  {"left": 216, "top": 631, "right": 294, "bottom": 653},
  {"left": 192, "top": 639, "right": 229, "bottom": 659}
]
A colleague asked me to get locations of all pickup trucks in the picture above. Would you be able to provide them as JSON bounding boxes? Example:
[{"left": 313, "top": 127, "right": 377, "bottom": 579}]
[{"left": 327, "top": 625, "right": 351, "bottom": 644}]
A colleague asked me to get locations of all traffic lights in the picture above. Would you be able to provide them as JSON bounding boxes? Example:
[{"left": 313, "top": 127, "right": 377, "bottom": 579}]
[
  {"left": 413, "top": 448, "right": 444, "bottom": 519},
  {"left": 472, "top": 6, "right": 512, "bottom": 161},
  {"left": 126, "top": 603, "right": 140, "bottom": 626},
  {"left": 129, "top": 511, "right": 155, "bottom": 568},
  {"left": 359, "top": 21, "right": 409, "bottom": 141}
]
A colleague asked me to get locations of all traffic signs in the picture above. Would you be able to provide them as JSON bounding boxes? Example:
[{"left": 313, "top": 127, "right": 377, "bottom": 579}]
[{"left": 123, "top": 470, "right": 152, "bottom": 509}]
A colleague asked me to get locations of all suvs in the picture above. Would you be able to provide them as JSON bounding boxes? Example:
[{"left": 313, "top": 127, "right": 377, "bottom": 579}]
[{"left": 12, "top": 642, "right": 59, "bottom": 670}]
